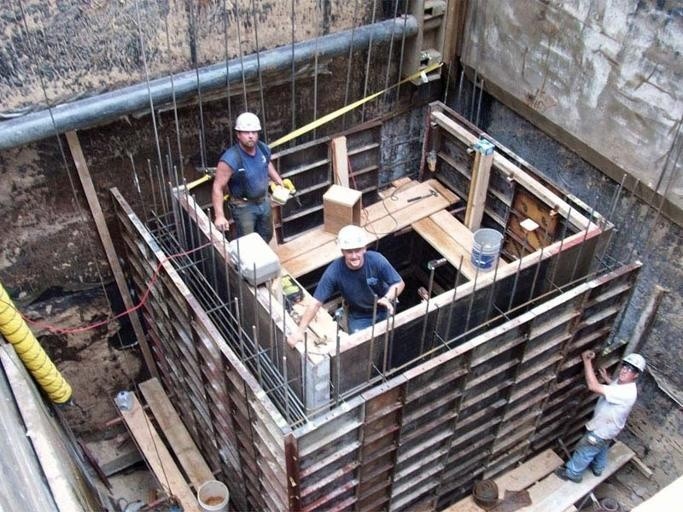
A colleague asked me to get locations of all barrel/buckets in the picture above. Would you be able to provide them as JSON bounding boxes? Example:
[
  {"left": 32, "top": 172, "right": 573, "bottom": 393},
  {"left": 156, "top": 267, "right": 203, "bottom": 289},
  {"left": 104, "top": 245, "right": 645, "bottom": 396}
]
[
  {"left": 197, "top": 481, "right": 230, "bottom": 512},
  {"left": 116, "top": 390, "right": 133, "bottom": 410},
  {"left": 471, "top": 228, "right": 503, "bottom": 273}
]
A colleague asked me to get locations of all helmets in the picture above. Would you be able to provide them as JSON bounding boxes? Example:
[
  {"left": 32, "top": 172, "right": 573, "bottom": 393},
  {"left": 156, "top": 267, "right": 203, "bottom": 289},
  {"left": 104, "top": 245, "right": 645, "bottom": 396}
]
[
  {"left": 621, "top": 353, "right": 646, "bottom": 374},
  {"left": 337, "top": 224, "right": 368, "bottom": 250},
  {"left": 234, "top": 112, "right": 262, "bottom": 132}
]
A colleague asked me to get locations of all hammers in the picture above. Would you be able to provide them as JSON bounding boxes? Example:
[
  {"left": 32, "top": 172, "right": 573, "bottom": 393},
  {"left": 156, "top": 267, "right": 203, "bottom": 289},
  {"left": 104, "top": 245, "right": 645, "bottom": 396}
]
[{"left": 407, "top": 189, "right": 438, "bottom": 202}]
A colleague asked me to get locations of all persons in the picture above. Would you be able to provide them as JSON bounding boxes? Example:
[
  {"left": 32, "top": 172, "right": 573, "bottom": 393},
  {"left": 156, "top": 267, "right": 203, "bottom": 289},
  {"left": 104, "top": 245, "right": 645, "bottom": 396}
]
[
  {"left": 286, "top": 225, "right": 406, "bottom": 351},
  {"left": 555, "top": 349, "right": 646, "bottom": 483},
  {"left": 212, "top": 112, "right": 284, "bottom": 245}
]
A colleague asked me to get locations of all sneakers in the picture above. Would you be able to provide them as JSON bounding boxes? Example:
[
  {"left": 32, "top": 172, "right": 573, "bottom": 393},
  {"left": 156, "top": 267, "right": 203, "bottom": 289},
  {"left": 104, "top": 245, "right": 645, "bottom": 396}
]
[
  {"left": 588, "top": 461, "right": 602, "bottom": 476},
  {"left": 555, "top": 466, "right": 583, "bottom": 483}
]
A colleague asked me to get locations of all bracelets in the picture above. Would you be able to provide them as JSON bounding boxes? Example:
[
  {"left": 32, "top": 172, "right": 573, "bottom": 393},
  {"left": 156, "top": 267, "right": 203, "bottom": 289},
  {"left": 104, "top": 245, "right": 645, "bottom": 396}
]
[{"left": 384, "top": 296, "right": 393, "bottom": 304}]
